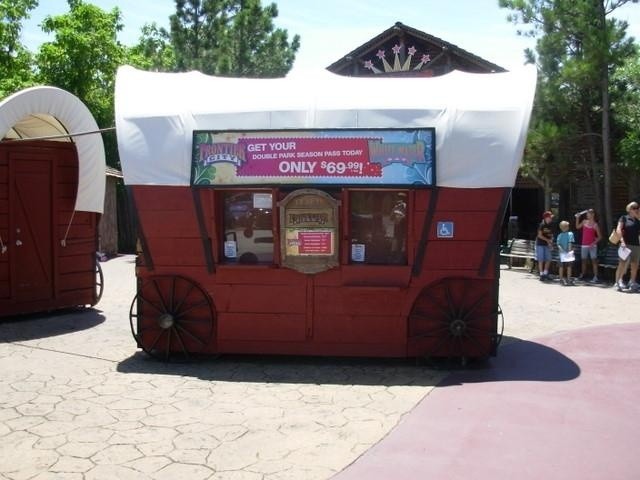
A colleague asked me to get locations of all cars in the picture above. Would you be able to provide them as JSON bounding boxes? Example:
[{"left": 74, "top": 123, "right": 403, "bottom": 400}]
[{"left": 224, "top": 200, "right": 387, "bottom": 250}]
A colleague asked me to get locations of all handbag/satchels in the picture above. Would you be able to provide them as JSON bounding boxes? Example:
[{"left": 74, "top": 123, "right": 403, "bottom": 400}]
[{"left": 609, "top": 229, "right": 620, "bottom": 245}]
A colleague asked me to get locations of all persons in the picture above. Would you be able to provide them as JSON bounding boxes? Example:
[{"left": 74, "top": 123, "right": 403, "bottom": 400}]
[
  {"left": 535, "top": 211, "right": 555, "bottom": 281},
  {"left": 389, "top": 192, "right": 407, "bottom": 262},
  {"left": 573, "top": 206, "right": 603, "bottom": 284},
  {"left": 616, "top": 202, "right": 640, "bottom": 294},
  {"left": 555, "top": 220, "right": 575, "bottom": 287}
]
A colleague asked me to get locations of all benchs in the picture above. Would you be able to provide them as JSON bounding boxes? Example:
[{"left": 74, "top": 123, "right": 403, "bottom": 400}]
[{"left": 499, "top": 238, "right": 619, "bottom": 285}]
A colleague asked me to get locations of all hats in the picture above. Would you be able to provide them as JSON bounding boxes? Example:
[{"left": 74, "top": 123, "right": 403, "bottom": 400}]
[{"left": 543, "top": 212, "right": 553, "bottom": 217}]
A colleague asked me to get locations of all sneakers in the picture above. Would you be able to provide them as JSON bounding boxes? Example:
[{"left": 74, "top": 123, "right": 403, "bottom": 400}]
[
  {"left": 560, "top": 274, "right": 599, "bottom": 286},
  {"left": 540, "top": 274, "right": 552, "bottom": 280},
  {"left": 616, "top": 280, "right": 640, "bottom": 290}
]
